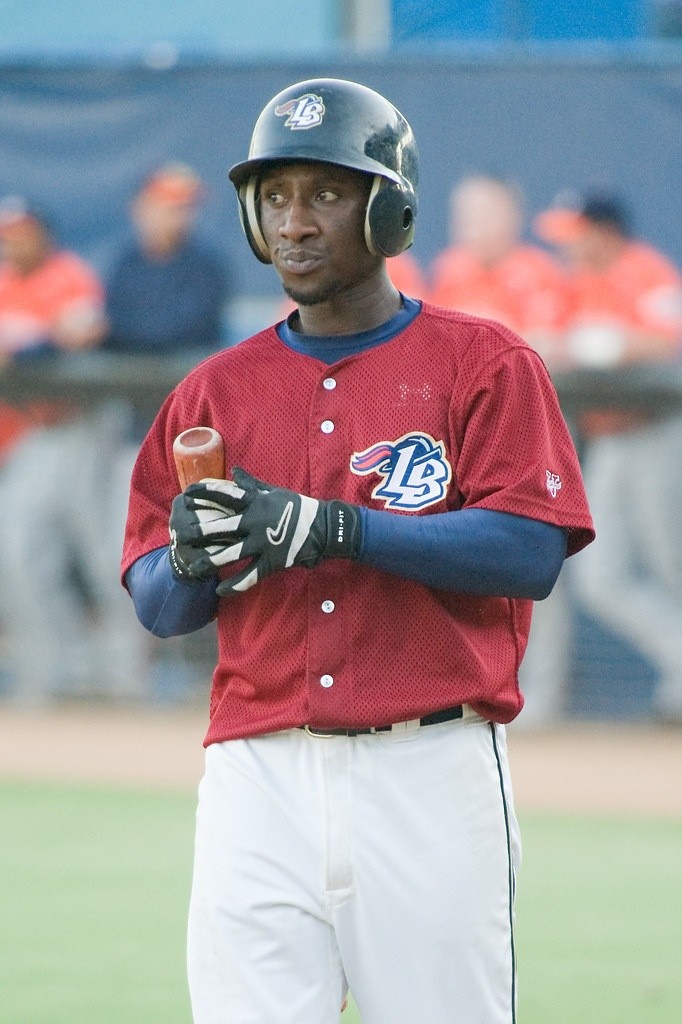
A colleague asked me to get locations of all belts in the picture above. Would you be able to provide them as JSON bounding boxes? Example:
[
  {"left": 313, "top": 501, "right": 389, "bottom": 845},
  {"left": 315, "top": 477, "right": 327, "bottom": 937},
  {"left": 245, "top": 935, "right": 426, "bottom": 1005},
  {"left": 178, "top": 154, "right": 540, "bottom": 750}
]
[{"left": 292, "top": 703, "right": 479, "bottom": 738}]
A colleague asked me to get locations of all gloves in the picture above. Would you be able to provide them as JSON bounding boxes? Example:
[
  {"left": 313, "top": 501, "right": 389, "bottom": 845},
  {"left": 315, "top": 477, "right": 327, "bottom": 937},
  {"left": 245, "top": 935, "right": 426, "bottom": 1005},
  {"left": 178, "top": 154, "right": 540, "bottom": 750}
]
[
  {"left": 184, "top": 466, "right": 362, "bottom": 597},
  {"left": 169, "top": 478, "right": 270, "bottom": 583}
]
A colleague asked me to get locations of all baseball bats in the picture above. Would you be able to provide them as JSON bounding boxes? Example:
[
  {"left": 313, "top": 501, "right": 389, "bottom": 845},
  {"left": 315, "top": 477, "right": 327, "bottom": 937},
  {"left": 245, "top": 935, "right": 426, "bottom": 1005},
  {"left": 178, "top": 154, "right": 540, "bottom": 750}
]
[{"left": 172, "top": 424, "right": 225, "bottom": 492}]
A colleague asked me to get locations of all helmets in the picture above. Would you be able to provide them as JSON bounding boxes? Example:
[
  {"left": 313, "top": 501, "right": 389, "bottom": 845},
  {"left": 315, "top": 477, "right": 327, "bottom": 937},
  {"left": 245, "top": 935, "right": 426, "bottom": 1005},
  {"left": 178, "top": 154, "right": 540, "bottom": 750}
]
[{"left": 228, "top": 78, "right": 420, "bottom": 264}]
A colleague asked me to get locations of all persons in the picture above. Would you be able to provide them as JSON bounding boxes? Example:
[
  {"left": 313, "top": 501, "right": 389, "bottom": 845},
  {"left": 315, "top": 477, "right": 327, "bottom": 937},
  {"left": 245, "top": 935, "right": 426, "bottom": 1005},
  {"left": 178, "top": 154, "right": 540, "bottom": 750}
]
[
  {"left": 121, "top": 78, "right": 595, "bottom": 1023},
  {"left": 0, "top": 157, "right": 681, "bottom": 728}
]
